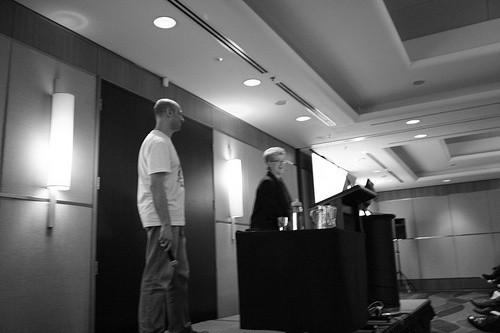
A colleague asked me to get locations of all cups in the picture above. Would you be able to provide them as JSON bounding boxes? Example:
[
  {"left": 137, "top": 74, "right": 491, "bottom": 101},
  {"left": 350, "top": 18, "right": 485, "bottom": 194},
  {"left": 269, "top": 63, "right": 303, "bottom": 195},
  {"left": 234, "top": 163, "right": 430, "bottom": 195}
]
[{"left": 277, "top": 216, "right": 288, "bottom": 230}]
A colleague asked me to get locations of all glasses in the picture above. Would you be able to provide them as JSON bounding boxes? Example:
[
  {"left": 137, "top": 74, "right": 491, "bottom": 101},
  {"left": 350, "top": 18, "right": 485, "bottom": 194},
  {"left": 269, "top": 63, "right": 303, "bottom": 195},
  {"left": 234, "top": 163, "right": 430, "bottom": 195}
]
[{"left": 271, "top": 159, "right": 287, "bottom": 164}]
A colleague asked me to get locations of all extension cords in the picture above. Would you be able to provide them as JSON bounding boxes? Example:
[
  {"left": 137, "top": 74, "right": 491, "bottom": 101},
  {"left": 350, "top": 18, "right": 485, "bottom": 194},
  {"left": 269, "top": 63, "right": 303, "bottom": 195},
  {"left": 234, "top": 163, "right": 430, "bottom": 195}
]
[{"left": 369, "top": 313, "right": 392, "bottom": 319}]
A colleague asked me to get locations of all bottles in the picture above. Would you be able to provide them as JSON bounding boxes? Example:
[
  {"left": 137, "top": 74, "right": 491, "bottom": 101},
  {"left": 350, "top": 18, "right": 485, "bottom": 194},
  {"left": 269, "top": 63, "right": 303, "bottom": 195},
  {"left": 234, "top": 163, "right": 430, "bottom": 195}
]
[{"left": 290, "top": 198, "right": 304, "bottom": 230}]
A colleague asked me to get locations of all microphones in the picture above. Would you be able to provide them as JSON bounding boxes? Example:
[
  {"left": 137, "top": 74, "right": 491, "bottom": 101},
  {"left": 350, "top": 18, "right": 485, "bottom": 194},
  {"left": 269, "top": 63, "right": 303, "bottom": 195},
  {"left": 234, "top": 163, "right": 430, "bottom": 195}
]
[{"left": 161, "top": 242, "right": 179, "bottom": 266}]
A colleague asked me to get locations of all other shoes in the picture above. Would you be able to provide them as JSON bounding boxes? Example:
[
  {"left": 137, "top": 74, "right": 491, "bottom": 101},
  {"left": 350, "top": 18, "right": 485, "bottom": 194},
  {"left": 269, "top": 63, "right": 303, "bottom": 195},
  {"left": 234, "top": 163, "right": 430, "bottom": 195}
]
[
  {"left": 487, "top": 279, "right": 496, "bottom": 286},
  {"left": 468, "top": 316, "right": 487, "bottom": 332},
  {"left": 470, "top": 299, "right": 490, "bottom": 308},
  {"left": 473, "top": 307, "right": 490, "bottom": 315},
  {"left": 483, "top": 274, "right": 494, "bottom": 280}
]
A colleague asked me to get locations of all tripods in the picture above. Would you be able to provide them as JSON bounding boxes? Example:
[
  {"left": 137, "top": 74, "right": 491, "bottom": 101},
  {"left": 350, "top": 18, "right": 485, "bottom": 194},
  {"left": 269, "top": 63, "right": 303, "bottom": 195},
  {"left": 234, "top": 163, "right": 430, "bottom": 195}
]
[{"left": 394, "top": 239, "right": 415, "bottom": 293}]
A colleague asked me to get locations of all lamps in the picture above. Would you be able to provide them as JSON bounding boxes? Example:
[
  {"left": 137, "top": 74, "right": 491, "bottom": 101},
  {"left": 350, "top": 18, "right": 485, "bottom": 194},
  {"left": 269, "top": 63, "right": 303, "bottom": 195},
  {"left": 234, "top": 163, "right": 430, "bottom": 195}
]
[
  {"left": 46, "top": 78, "right": 75, "bottom": 228},
  {"left": 227, "top": 144, "right": 243, "bottom": 241}
]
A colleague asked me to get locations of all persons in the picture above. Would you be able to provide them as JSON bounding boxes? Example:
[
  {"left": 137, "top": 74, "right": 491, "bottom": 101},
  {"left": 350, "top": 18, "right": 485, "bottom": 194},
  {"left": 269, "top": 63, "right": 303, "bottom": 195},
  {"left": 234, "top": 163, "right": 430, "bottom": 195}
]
[
  {"left": 251, "top": 147, "right": 293, "bottom": 230},
  {"left": 137, "top": 98, "right": 207, "bottom": 333},
  {"left": 467, "top": 264, "right": 500, "bottom": 333}
]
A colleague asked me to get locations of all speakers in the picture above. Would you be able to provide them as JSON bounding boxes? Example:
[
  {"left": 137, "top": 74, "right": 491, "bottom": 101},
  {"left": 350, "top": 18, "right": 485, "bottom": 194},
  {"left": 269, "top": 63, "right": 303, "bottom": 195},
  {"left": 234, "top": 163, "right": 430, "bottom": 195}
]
[{"left": 391, "top": 218, "right": 406, "bottom": 239}]
